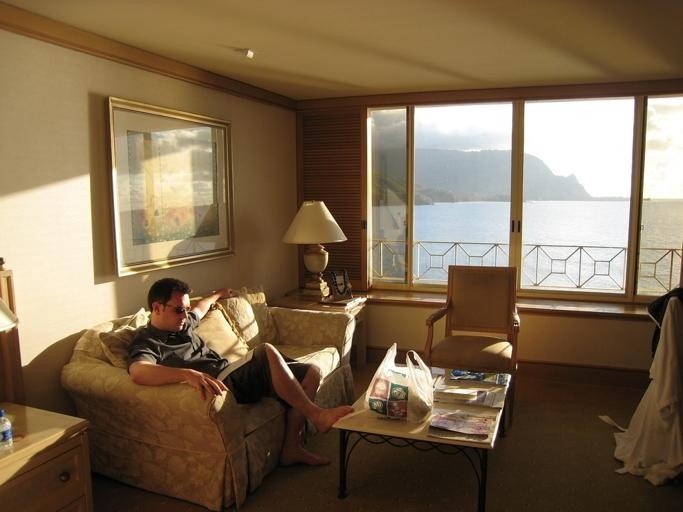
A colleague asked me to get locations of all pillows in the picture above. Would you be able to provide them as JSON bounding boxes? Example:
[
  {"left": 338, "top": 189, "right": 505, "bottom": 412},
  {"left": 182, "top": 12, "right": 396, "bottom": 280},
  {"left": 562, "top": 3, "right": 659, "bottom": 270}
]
[{"left": 95, "top": 292, "right": 280, "bottom": 372}]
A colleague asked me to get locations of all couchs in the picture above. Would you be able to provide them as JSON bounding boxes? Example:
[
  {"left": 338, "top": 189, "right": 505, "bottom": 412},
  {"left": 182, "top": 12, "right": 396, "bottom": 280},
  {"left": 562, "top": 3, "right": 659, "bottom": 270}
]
[{"left": 56, "top": 287, "right": 356, "bottom": 512}]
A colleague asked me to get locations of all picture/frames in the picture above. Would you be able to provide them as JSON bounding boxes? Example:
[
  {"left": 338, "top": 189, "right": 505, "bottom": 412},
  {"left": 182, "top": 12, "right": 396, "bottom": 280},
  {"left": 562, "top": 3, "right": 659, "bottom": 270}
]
[
  {"left": 105, "top": 94, "right": 236, "bottom": 279},
  {"left": 329, "top": 269, "right": 353, "bottom": 303}
]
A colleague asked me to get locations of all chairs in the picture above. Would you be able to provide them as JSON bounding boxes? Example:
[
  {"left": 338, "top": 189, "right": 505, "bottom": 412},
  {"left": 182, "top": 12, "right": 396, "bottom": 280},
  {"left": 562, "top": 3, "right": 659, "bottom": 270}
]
[{"left": 424, "top": 264, "right": 520, "bottom": 419}]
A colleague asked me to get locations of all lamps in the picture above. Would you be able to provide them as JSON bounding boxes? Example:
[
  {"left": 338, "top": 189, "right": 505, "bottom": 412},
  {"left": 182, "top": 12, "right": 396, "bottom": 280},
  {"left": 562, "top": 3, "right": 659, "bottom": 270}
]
[
  {"left": 0, "top": 297, "right": 19, "bottom": 333},
  {"left": 281, "top": 199, "right": 348, "bottom": 301}
]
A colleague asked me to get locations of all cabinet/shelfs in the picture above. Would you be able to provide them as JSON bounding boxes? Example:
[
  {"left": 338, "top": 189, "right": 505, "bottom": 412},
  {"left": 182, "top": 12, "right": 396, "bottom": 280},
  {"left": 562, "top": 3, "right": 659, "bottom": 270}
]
[
  {"left": 267, "top": 292, "right": 366, "bottom": 374},
  {"left": 0, "top": 399, "right": 96, "bottom": 512},
  {"left": 0, "top": 269, "right": 26, "bottom": 407}
]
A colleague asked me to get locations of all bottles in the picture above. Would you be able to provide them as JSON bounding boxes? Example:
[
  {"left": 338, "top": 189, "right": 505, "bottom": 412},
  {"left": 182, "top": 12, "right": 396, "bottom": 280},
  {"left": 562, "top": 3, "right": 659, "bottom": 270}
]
[{"left": 0, "top": 409, "right": 14, "bottom": 453}]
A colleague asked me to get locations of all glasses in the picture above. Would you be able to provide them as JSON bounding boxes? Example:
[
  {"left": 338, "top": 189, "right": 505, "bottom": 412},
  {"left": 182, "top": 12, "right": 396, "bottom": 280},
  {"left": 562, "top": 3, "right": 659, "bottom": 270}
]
[{"left": 160, "top": 302, "right": 192, "bottom": 314}]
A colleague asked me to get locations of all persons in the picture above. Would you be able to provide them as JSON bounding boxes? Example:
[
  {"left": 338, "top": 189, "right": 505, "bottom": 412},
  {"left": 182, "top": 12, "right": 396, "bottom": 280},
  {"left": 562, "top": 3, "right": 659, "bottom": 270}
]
[{"left": 128, "top": 278, "right": 354, "bottom": 466}]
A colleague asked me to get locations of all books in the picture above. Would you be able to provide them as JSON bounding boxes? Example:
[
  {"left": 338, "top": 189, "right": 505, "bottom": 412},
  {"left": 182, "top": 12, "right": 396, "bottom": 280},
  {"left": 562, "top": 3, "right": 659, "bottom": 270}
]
[{"left": 428, "top": 373, "right": 477, "bottom": 400}]
